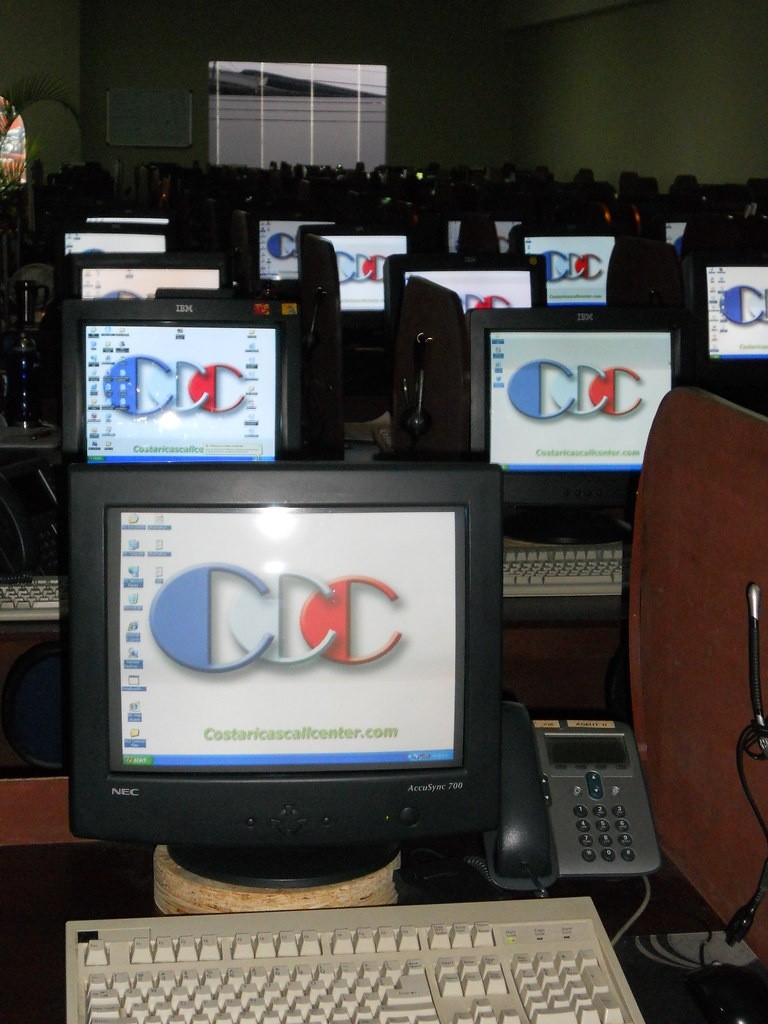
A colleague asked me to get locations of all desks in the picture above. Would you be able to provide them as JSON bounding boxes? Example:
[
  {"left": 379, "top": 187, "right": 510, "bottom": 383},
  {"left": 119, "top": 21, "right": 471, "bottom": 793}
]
[{"left": 0, "top": 234, "right": 768, "bottom": 1024}]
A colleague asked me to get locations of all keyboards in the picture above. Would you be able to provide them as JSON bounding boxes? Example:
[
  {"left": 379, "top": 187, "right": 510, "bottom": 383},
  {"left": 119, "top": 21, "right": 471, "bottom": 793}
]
[
  {"left": 0, "top": 574, "right": 60, "bottom": 622},
  {"left": 499, "top": 541, "right": 623, "bottom": 596},
  {"left": 66, "top": 896, "right": 645, "bottom": 1024}
]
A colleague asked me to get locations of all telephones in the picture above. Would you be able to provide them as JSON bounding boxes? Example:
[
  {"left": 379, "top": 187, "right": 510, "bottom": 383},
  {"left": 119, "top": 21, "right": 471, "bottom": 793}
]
[{"left": 480, "top": 697, "right": 663, "bottom": 891}]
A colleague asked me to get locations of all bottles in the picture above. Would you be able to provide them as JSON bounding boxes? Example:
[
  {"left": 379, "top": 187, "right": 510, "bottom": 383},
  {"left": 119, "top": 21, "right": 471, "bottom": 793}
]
[
  {"left": 7, "top": 332, "right": 44, "bottom": 427},
  {"left": 260, "top": 280, "right": 278, "bottom": 301}
]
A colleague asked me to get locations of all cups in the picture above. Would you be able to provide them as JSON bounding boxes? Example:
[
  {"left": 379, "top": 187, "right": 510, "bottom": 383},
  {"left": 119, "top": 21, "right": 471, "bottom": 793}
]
[{"left": 13, "top": 280, "right": 50, "bottom": 329}]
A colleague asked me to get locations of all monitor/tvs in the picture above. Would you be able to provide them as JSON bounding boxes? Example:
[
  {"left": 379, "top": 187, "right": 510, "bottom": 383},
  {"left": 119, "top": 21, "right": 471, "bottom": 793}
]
[{"left": 0, "top": 156, "right": 768, "bottom": 883}]
